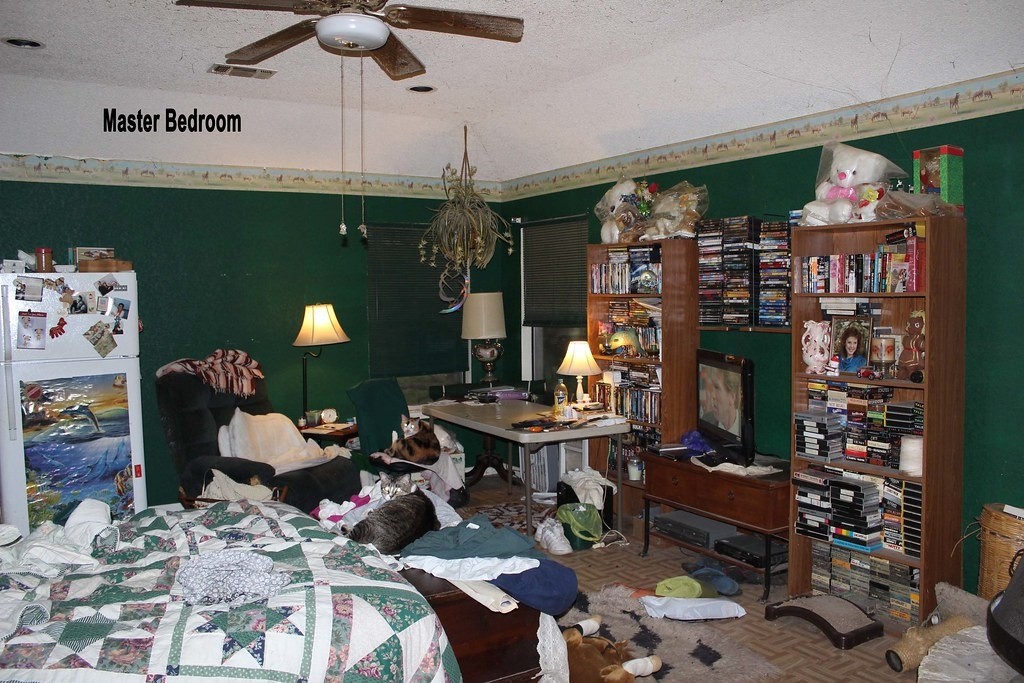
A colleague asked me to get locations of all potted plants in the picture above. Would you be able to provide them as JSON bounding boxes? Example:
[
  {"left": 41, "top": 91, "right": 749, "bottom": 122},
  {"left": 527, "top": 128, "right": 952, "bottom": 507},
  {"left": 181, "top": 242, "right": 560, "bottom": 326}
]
[{"left": 419, "top": 126, "right": 514, "bottom": 276}]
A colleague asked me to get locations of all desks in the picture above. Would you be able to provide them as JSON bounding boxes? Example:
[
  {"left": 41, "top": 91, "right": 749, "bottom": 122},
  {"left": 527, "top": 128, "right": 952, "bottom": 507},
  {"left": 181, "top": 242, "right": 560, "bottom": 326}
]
[
  {"left": 638, "top": 447, "right": 790, "bottom": 603},
  {"left": 423, "top": 400, "right": 630, "bottom": 539}
]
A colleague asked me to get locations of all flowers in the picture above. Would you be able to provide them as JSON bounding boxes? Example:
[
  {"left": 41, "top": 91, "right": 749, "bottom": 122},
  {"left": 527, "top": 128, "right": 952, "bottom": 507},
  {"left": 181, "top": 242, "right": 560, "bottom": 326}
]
[{"left": 633, "top": 178, "right": 661, "bottom": 216}]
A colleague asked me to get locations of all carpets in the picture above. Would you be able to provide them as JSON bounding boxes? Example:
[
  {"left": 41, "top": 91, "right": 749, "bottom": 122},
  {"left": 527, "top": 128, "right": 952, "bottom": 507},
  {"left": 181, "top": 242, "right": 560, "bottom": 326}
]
[
  {"left": 560, "top": 582, "right": 785, "bottom": 683},
  {"left": 453, "top": 503, "right": 558, "bottom": 537}
]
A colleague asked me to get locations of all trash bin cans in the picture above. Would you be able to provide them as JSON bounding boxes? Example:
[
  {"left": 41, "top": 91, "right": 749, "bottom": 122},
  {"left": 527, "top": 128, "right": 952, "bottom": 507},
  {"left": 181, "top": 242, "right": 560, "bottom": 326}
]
[{"left": 558, "top": 503, "right": 597, "bottom": 550}]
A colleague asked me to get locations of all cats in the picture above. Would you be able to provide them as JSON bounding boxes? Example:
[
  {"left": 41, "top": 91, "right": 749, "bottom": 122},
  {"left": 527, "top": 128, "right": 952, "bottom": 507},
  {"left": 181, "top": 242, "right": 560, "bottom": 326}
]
[
  {"left": 326, "top": 471, "right": 442, "bottom": 556},
  {"left": 383, "top": 413, "right": 441, "bottom": 466}
]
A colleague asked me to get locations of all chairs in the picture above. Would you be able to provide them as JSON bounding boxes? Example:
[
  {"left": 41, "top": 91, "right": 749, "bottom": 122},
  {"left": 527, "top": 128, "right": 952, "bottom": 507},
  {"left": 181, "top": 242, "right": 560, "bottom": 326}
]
[{"left": 345, "top": 377, "right": 426, "bottom": 484}]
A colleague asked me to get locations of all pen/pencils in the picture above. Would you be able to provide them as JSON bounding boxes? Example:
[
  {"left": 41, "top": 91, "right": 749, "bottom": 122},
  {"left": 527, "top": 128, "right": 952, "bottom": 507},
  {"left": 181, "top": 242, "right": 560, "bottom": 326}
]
[{"left": 324, "top": 426, "right": 335, "bottom": 428}]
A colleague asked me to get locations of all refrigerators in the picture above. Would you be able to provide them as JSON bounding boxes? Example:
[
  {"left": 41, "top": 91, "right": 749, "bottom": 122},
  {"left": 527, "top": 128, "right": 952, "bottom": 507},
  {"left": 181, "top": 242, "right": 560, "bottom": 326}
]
[{"left": 0, "top": 272, "right": 148, "bottom": 541}]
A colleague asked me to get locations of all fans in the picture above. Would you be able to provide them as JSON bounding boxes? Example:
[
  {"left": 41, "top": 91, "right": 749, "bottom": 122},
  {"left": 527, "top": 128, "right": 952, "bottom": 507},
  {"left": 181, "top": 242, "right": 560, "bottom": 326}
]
[{"left": 175, "top": 0, "right": 523, "bottom": 82}]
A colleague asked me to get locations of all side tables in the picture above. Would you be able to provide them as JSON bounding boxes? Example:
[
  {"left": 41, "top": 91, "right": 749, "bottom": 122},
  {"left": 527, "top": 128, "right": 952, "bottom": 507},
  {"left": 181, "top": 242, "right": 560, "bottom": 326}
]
[{"left": 294, "top": 421, "right": 358, "bottom": 451}]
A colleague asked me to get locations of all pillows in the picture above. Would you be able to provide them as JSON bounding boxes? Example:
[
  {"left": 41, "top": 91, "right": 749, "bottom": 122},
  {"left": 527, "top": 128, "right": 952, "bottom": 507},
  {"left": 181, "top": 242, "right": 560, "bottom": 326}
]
[{"left": 218, "top": 406, "right": 302, "bottom": 462}]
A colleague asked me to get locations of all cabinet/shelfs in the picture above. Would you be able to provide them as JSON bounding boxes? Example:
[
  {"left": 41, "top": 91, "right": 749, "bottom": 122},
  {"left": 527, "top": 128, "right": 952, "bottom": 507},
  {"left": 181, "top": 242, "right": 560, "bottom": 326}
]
[
  {"left": 788, "top": 217, "right": 967, "bottom": 627},
  {"left": 585, "top": 238, "right": 700, "bottom": 492}
]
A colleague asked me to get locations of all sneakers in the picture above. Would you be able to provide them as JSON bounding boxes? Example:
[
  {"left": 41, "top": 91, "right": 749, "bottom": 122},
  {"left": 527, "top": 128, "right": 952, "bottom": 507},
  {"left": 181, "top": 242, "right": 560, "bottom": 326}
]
[
  {"left": 541, "top": 524, "right": 573, "bottom": 555},
  {"left": 535, "top": 519, "right": 559, "bottom": 541}
]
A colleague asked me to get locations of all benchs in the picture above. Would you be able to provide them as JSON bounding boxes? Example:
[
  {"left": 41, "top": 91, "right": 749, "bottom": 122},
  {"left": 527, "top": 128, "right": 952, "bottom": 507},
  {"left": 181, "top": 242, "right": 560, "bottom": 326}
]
[{"left": 312, "top": 505, "right": 552, "bottom": 682}]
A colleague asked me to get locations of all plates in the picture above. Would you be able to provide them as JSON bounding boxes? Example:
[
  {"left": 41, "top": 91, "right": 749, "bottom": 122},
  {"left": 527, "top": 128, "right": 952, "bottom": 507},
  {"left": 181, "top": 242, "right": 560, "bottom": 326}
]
[{"left": 53, "top": 265, "right": 77, "bottom": 270}]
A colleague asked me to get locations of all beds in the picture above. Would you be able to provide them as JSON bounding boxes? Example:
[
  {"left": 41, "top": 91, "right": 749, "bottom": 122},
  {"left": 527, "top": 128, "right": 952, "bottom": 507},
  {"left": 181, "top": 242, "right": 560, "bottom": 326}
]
[{"left": 0, "top": 493, "right": 462, "bottom": 682}]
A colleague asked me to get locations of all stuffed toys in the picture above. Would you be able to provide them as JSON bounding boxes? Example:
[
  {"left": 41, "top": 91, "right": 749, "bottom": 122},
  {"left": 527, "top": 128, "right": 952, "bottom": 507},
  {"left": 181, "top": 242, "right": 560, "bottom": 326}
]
[
  {"left": 804, "top": 144, "right": 888, "bottom": 226},
  {"left": 920, "top": 153, "right": 940, "bottom": 195},
  {"left": 596, "top": 181, "right": 702, "bottom": 243}
]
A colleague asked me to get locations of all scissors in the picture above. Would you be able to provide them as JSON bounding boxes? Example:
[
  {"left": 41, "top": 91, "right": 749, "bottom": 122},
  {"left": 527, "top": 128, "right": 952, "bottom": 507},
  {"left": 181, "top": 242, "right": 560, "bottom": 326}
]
[{"left": 506, "top": 426, "right": 542, "bottom": 432}]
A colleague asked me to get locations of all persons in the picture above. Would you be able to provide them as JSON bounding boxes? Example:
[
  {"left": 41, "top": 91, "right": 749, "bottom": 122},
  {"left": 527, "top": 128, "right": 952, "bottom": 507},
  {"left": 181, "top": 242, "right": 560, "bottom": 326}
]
[
  {"left": 70, "top": 294, "right": 87, "bottom": 314},
  {"left": 113, "top": 303, "right": 124, "bottom": 318},
  {"left": 98, "top": 281, "right": 110, "bottom": 295},
  {"left": 702, "top": 369, "right": 741, "bottom": 434},
  {"left": 837, "top": 328, "right": 866, "bottom": 372}
]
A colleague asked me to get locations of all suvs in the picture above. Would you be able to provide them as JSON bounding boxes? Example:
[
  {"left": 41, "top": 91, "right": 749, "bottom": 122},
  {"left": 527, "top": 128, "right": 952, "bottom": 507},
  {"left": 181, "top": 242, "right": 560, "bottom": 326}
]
[{"left": 857, "top": 366, "right": 884, "bottom": 380}]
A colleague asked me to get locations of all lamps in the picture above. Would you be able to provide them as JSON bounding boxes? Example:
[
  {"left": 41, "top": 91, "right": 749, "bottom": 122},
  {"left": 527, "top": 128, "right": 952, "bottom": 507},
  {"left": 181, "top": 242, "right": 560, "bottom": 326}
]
[
  {"left": 461, "top": 291, "right": 506, "bottom": 388},
  {"left": 557, "top": 340, "right": 599, "bottom": 403},
  {"left": 290, "top": 304, "right": 349, "bottom": 419},
  {"left": 316, "top": 14, "right": 389, "bottom": 53}
]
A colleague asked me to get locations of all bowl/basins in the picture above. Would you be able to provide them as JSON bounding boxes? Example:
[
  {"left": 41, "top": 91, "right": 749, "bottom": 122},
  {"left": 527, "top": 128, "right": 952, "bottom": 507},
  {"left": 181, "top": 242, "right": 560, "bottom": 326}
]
[{"left": 306, "top": 410, "right": 321, "bottom": 424}]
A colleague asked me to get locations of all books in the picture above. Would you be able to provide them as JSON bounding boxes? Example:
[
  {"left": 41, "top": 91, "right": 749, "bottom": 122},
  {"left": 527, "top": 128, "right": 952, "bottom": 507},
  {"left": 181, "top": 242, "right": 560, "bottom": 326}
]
[{"left": 800, "top": 223, "right": 927, "bottom": 293}]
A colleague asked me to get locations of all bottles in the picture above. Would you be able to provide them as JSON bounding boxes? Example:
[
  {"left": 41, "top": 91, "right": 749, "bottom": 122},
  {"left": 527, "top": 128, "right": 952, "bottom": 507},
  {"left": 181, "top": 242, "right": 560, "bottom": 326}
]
[
  {"left": 628, "top": 456, "right": 642, "bottom": 481},
  {"left": 554, "top": 379, "right": 568, "bottom": 415},
  {"left": 34, "top": 248, "right": 51, "bottom": 272}
]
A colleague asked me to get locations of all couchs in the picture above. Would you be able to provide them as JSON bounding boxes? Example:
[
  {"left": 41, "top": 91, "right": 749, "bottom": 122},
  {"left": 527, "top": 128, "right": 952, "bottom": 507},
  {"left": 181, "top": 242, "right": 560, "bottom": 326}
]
[{"left": 158, "top": 358, "right": 355, "bottom": 508}]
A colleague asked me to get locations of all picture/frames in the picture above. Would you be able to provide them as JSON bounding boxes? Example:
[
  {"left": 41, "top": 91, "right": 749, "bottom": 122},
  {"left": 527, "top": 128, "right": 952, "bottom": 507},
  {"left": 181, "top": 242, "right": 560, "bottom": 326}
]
[{"left": 825, "top": 315, "right": 877, "bottom": 374}]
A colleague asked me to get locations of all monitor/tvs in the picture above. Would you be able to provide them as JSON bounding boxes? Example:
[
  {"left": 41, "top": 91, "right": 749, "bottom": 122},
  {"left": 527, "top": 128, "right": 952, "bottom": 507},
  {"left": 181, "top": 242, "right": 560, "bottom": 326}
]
[{"left": 694, "top": 347, "right": 756, "bottom": 468}]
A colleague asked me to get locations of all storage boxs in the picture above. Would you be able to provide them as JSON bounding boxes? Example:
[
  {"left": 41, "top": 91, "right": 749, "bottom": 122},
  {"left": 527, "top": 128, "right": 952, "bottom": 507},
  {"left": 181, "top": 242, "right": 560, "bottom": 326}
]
[
  {"left": 78, "top": 260, "right": 133, "bottom": 272},
  {"left": 913, "top": 144, "right": 965, "bottom": 213}
]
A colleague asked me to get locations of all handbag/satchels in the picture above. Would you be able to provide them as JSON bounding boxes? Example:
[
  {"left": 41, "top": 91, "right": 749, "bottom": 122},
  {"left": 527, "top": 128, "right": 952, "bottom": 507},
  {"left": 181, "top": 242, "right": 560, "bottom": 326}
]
[{"left": 180, "top": 456, "right": 287, "bottom": 509}]
